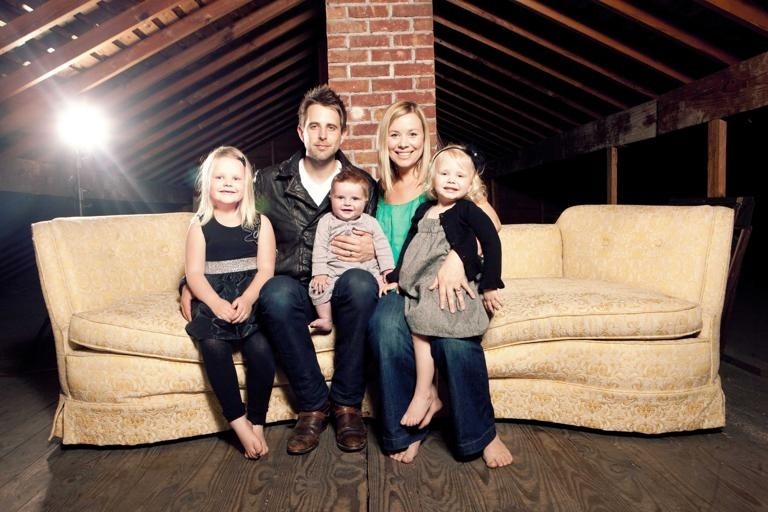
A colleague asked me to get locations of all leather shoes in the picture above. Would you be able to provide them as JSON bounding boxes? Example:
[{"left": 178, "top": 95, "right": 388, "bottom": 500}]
[{"left": 287, "top": 405, "right": 368, "bottom": 454}]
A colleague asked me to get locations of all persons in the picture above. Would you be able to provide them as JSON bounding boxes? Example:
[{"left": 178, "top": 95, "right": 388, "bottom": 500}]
[
  {"left": 178, "top": 86, "right": 379, "bottom": 457},
  {"left": 180, "top": 144, "right": 277, "bottom": 460},
  {"left": 307, "top": 167, "right": 397, "bottom": 333},
  {"left": 358, "top": 100, "right": 514, "bottom": 470},
  {"left": 388, "top": 142, "right": 506, "bottom": 430}
]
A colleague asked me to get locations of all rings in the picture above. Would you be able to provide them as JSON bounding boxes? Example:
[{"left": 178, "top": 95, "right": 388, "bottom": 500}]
[
  {"left": 349, "top": 250, "right": 353, "bottom": 258},
  {"left": 455, "top": 286, "right": 462, "bottom": 293}
]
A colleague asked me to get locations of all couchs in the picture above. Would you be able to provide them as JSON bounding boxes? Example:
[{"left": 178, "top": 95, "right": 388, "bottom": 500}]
[{"left": 28, "top": 203, "right": 735, "bottom": 446}]
